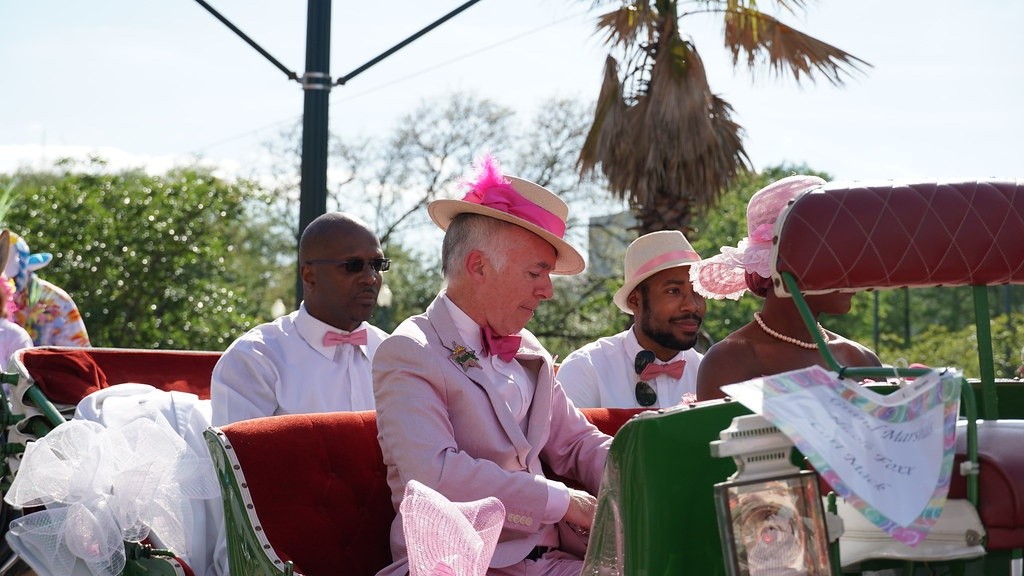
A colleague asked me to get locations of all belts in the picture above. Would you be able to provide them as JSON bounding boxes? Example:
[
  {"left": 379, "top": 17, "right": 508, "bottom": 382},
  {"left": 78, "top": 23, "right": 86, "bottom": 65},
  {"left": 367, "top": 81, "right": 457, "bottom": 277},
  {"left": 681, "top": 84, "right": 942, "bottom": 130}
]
[{"left": 525, "top": 546, "right": 561, "bottom": 562}]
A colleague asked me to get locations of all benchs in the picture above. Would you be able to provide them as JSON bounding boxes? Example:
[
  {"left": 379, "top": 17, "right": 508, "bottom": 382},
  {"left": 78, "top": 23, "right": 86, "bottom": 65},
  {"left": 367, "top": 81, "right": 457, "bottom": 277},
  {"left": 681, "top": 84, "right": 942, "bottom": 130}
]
[
  {"left": 204, "top": 405, "right": 667, "bottom": 576},
  {"left": 771, "top": 179, "right": 1024, "bottom": 576},
  {"left": 11, "top": 345, "right": 225, "bottom": 576}
]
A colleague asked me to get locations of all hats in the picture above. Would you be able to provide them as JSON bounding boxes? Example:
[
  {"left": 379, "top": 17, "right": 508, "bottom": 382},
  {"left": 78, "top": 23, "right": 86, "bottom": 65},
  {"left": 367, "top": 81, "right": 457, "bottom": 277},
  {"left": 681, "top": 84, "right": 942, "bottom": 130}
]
[
  {"left": 427, "top": 150, "right": 585, "bottom": 275},
  {"left": 613, "top": 231, "right": 703, "bottom": 315},
  {"left": 688, "top": 175, "right": 828, "bottom": 301},
  {"left": 0, "top": 229, "right": 53, "bottom": 293}
]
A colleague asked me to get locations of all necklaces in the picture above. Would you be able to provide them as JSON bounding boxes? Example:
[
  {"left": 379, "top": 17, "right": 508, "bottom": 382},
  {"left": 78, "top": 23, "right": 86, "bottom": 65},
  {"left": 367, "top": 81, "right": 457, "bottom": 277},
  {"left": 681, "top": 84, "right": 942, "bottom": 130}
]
[{"left": 754, "top": 312, "right": 829, "bottom": 350}]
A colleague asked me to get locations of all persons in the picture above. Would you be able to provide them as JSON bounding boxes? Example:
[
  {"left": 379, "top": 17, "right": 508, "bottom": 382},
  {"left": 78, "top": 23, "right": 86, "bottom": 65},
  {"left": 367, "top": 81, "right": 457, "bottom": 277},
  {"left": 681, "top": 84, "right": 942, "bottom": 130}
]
[
  {"left": 697, "top": 175, "right": 886, "bottom": 402},
  {"left": 211, "top": 212, "right": 390, "bottom": 429},
  {"left": 557, "top": 230, "right": 708, "bottom": 410},
  {"left": 372, "top": 176, "right": 617, "bottom": 576},
  {"left": 0, "top": 277, "right": 34, "bottom": 395}
]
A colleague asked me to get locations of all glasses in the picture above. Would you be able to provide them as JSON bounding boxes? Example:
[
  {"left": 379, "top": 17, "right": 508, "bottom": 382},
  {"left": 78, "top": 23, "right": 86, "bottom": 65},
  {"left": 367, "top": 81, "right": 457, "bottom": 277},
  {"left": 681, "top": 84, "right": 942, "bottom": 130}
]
[
  {"left": 635, "top": 349, "right": 657, "bottom": 406},
  {"left": 305, "top": 256, "right": 392, "bottom": 272}
]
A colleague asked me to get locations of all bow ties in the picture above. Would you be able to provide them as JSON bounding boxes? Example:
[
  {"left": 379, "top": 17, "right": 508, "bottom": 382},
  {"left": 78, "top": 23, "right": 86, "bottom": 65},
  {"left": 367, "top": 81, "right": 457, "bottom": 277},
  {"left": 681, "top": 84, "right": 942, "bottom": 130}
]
[
  {"left": 479, "top": 327, "right": 522, "bottom": 363},
  {"left": 323, "top": 328, "right": 367, "bottom": 346},
  {"left": 641, "top": 360, "right": 686, "bottom": 380}
]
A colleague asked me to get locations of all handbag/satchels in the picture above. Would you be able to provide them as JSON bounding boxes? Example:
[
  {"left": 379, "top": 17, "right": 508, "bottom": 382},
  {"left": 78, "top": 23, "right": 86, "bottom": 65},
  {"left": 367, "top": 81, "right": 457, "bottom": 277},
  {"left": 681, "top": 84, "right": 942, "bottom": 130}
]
[
  {"left": 821, "top": 495, "right": 987, "bottom": 568},
  {"left": 558, "top": 520, "right": 590, "bottom": 559}
]
